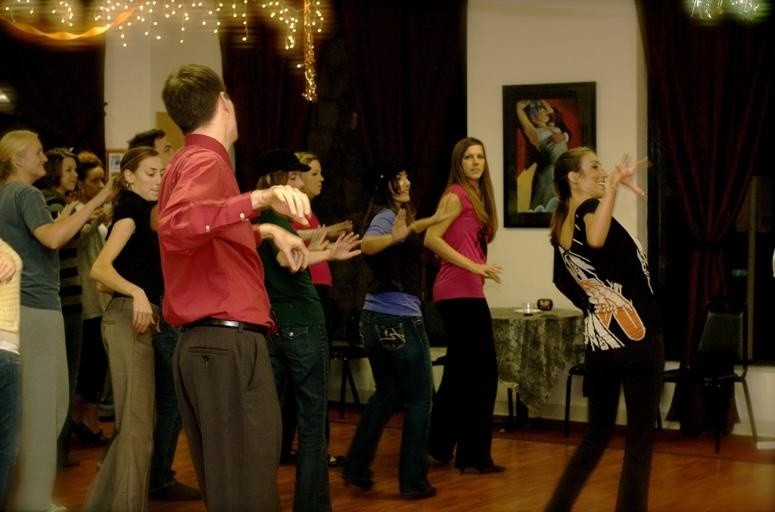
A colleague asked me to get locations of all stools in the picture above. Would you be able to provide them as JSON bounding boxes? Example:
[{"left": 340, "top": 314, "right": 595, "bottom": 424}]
[{"left": 331, "top": 348, "right": 365, "bottom": 412}]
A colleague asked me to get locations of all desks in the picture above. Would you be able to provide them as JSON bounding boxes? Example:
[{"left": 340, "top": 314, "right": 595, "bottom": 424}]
[{"left": 487, "top": 307, "right": 584, "bottom": 424}]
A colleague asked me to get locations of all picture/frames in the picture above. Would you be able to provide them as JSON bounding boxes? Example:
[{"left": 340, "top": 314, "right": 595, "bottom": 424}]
[{"left": 501, "top": 79, "right": 598, "bottom": 230}]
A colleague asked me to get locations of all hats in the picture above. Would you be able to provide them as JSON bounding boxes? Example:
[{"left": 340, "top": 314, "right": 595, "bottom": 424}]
[{"left": 258, "top": 150, "right": 311, "bottom": 173}]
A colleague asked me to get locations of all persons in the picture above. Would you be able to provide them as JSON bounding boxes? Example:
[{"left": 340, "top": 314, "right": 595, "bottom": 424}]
[
  {"left": 424, "top": 136, "right": 508, "bottom": 474},
  {"left": 1, "top": 64, "right": 363, "bottom": 511},
  {"left": 516, "top": 98, "right": 568, "bottom": 213},
  {"left": 341, "top": 166, "right": 450, "bottom": 502},
  {"left": 549, "top": 147, "right": 665, "bottom": 511}
]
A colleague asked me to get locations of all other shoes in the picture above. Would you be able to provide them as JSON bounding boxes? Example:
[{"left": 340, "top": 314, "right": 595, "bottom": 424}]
[
  {"left": 429, "top": 437, "right": 453, "bottom": 464},
  {"left": 328, "top": 454, "right": 346, "bottom": 467},
  {"left": 343, "top": 473, "right": 375, "bottom": 491},
  {"left": 76, "top": 421, "right": 113, "bottom": 445},
  {"left": 147, "top": 481, "right": 203, "bottom": 500},
  {"left": 277, "top": 449, "right": 297, "bottom": 465},
  {"left": 399, "top": 488, "right": 436, "bottom": 499}
]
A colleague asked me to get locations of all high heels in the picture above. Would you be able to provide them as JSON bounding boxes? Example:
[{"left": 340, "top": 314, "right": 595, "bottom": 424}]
[{"left": 459, "top": 463, "right": 504, "bottom": 474}]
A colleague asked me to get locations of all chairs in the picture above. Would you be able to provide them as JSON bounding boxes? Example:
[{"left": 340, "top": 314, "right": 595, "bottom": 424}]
[{"left": 660, "top": 301, "right": 756, "bottom": 452}]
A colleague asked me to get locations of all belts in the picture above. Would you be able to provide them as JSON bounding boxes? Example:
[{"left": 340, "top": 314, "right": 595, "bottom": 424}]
[{"left": 178, "top": 316, "right": 268, "bottom": 339}]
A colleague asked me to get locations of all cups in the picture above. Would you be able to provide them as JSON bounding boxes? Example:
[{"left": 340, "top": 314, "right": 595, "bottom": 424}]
[{"left": 522, "top": 302, "right": 535, "bottom": 316}]
